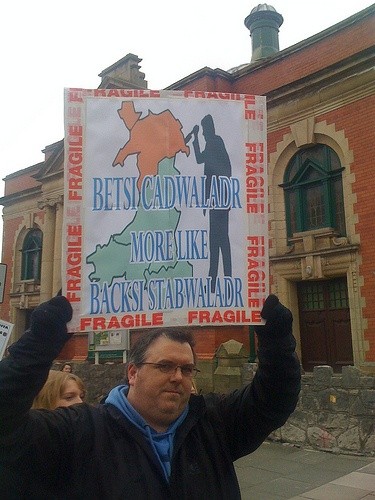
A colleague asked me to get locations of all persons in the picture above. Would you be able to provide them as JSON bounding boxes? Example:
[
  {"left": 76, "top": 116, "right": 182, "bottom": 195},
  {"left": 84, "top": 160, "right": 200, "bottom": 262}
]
[
  {"left": 61, "top": 363, "right": 75, "bottom": 373},
  {"left": 1, "top": 287, "right": 300, "bottom": 499},
  {"left": 33, "top": 370, "right": 84, "bottom": 411}
]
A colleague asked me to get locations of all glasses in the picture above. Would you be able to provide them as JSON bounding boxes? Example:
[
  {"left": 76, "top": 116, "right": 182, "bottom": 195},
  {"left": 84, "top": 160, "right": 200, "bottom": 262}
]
[{"left": 135, "top": 361, "right": 200, "bottom": 377}]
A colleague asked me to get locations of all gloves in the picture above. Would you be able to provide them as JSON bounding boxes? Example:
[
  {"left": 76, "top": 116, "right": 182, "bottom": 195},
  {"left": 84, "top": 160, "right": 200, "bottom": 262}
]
[
  {"left": 32, "top": 288, "right": 74, "bottom": 345},
  {"left": 255, "top": 294, "right": 293, "bottom": 339}
]
